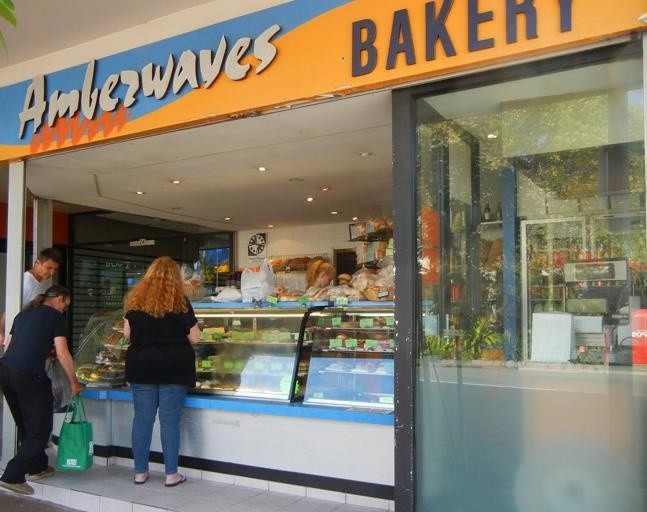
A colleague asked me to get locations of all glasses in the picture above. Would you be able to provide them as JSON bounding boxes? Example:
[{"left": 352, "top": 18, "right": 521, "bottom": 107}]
[{"left": 63, "top": 298, "right": 69, "bottom": 311}]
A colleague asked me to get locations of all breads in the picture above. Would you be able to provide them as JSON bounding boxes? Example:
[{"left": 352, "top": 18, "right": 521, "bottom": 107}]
[{"left": 75, "top": 215, "right": 394, "bottom": 393}]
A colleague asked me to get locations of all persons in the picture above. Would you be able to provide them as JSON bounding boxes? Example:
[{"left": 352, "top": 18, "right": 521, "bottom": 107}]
[
  {"left": 0, "top": 284, "right": 82, "bottom": 496},
  {"left": 301, "top": 259, "right": 337, "bottom": 296},
  {"left": 0, "top": 247, "right": 62, "bottom": 356},
  {"left": 120, "top": 256, "right": 202, "bottom": 487}
]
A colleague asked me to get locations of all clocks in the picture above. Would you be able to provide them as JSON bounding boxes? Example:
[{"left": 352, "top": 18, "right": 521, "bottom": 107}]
[{"left": 248, "top": 233, "right": 268, "bottom": 259}]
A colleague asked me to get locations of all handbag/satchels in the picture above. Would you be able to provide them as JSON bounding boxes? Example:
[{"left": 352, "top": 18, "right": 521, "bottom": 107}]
[{"left": 55, "top": 421, "right": 93, "bottom": 471}]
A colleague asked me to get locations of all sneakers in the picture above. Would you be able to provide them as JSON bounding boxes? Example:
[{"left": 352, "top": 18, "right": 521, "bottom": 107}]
[
  {"left": 0, "top": 481, "right": 34, "bottom": 495},
  {"left": 29, "top": 465, "right": 55, "bottom": 480}
]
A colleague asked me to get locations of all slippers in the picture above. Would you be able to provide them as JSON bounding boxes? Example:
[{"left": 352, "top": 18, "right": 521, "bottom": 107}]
[
  {"left": 135, "top": 473, "right": 149, "bottom": 484},
  {"left": 165, "top": 474, "right": 185, "bottom": 486}
]
